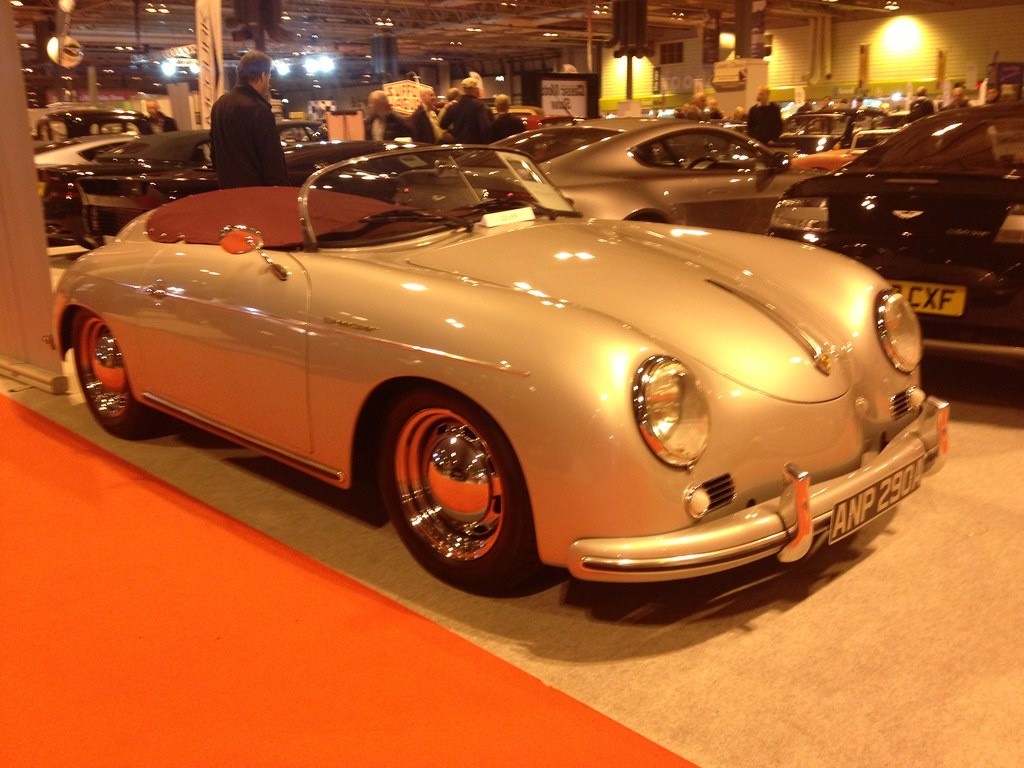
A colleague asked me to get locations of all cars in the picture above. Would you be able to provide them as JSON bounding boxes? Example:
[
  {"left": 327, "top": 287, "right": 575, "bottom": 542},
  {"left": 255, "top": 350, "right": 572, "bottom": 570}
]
[
  {"left": 397, "top": 116, "right": 845, "bottom": 244},
  {"left": 25, "top": 100, "right": 576, "bottom": 246},
  {"left": 769, "top": 94, "right": 1024, "bottom": 377},
  {"left": 779, "top": 126, "right": 908, "bottom": 178}
]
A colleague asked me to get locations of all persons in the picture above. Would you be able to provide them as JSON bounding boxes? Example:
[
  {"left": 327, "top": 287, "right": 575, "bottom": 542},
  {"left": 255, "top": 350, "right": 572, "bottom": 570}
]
[
  {"left": 821, "top": 96, "right": 831, "bottom": 110},
  {"left": 987, "top": 84, "right": 1001, "bottom": 104},
  {"left": 906, "top": 85, "right": 934, "bottom": 123},
  {"left": 673, "top": 93, "right": 744, "bottom": 122},
  {"left": 364, "top": 77, "right": 526, "bottom": 146},
  {"left": 746, "top": 85, "right": 783, "bottom": 146},
  {"left": 941, "top": 87, "right": 973, "bottom": 110},
  {"left": 797, "top": 97, "right": 814, "bottom": 112},
  {"left": 142, "top": 100, "right": 178, "bottom": 135},
  {"left": 209, "top": 50, "right": 289, "bottom": 189}
]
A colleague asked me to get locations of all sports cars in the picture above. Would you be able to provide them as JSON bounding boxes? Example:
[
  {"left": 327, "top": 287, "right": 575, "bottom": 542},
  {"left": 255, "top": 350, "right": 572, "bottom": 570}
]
[{"left": 47, "top": 141, "right": 952, "bottom": 598}]
